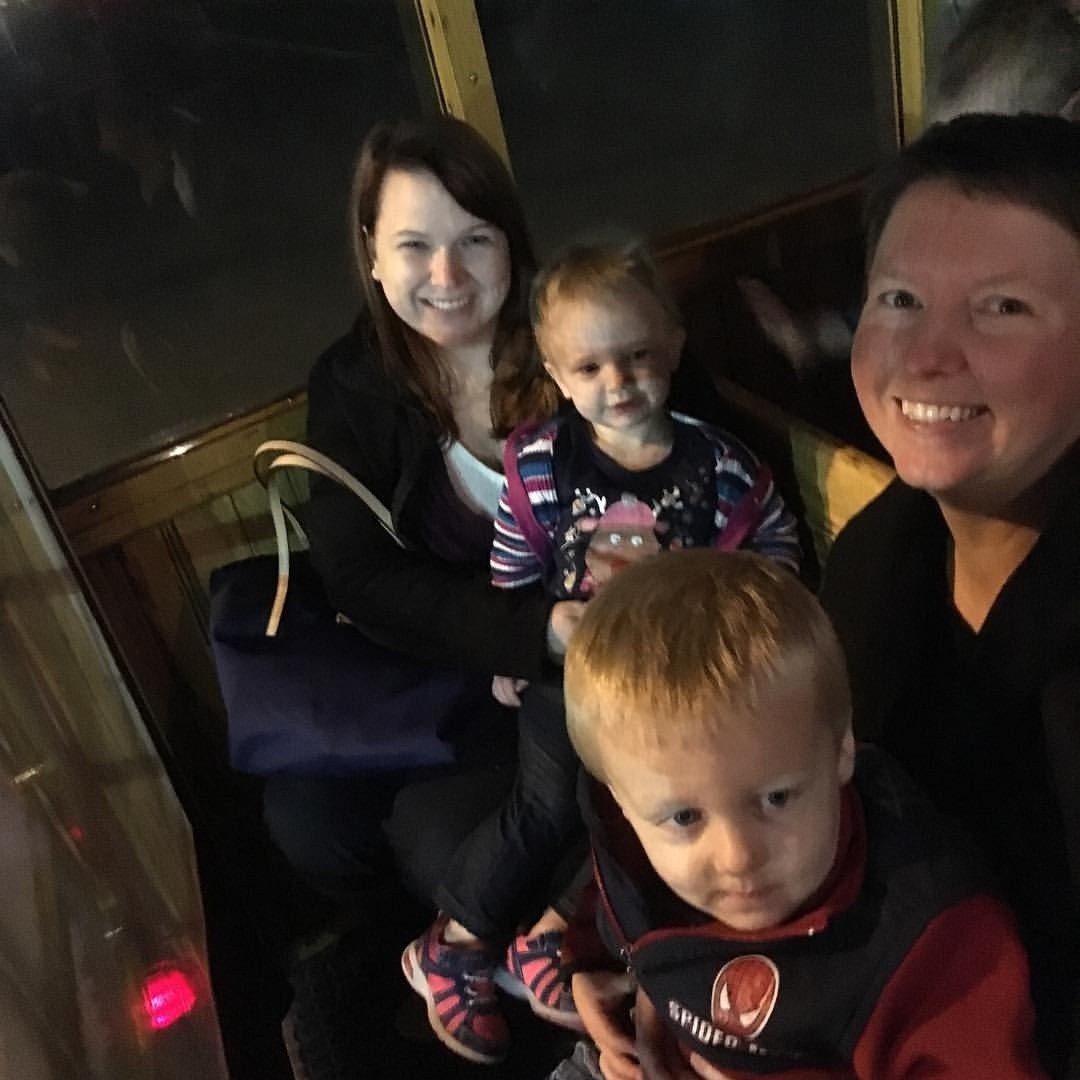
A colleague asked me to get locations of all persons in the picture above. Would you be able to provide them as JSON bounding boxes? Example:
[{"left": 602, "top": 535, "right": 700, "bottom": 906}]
[
  {"left": 808, "top": 110, "right": 1080, "bottom": 1078},
  {"left": 528, "top": 544, "right": 1053, "bottom": 1078},
  {"left": 301, "top": 112, "right": 591, "bottom": 938},
  {"left": 401, "top": 227, "right": 808, "bottom": 1069}
]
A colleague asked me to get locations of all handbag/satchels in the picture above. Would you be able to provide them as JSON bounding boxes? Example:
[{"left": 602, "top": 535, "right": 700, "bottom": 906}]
[{"left": 207, "top": 440, "right": 496, "bottom": 782}]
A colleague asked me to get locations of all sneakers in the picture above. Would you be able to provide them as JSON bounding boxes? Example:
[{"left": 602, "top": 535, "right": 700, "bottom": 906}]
[
  {"left": 401, "top": 913, "right": 516, "bottom": 1062},
  {"left": 493, "top": 925, "right": 590, "bottom": 1036}
]
